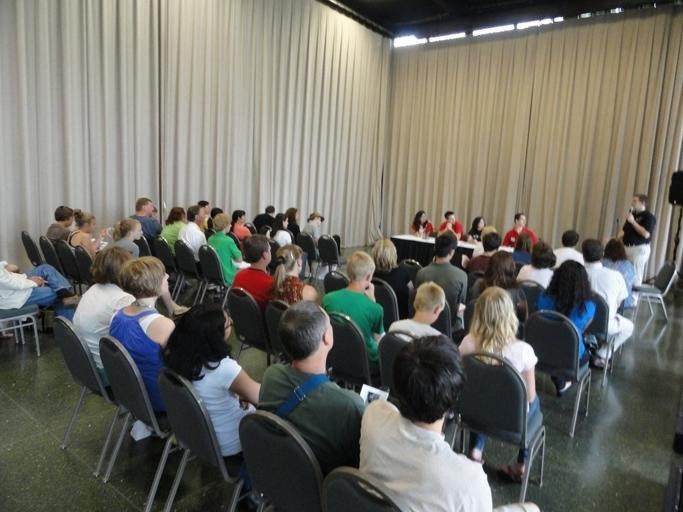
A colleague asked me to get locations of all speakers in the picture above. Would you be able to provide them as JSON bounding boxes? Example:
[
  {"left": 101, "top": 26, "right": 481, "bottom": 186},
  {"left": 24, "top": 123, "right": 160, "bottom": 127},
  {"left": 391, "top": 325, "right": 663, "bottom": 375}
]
[{"left": 668, "top": 170, "right": 683, "bottom": 205}]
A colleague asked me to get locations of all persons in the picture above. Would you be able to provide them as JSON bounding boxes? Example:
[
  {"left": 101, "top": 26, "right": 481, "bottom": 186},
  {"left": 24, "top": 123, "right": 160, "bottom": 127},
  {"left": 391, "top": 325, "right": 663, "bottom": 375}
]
[
  {"left": 254, "top": 299, "right": 366, "bottom": 479},
  {"left": 357, "top": 333, "right": 543, "bottom": 512},
  {"left": 157, "top": 300, "right": 267, "bottom": 512},
  {"left": 47, "top": 196, "right": 641, "bottom": 420},
  {"left": 605, "top": 192, "right": 658, "bottom": 312},
  {"left": 458, "top": 285, "right": 544, "bottom": 486},
  {"left": 0, "top": 259, "right": 83, "bottom": 323},
  {"left": 105, "top": 251, "right": 178, "bottom": 437}
]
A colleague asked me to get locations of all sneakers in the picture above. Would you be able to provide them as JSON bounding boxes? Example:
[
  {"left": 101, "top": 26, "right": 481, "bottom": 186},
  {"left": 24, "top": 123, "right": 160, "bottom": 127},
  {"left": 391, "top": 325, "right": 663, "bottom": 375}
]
[
  {"left": 169, "top": 305, "right": 192, "bottom": 319},
  {"left": 63, "top": 294, "right": 82, "bottom": 306}
]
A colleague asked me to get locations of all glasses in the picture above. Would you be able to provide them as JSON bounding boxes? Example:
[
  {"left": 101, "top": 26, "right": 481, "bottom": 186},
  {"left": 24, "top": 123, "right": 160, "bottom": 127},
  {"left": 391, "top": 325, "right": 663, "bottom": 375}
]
[{"left": 225, "top": 317, "right": 234, "bottom": 330}]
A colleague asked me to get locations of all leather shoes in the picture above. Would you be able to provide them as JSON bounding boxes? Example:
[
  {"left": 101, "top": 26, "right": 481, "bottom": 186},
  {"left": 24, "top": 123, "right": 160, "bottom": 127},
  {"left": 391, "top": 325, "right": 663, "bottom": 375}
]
[{"left": 500, "top": 463, "right": 523, "bottom": 485}]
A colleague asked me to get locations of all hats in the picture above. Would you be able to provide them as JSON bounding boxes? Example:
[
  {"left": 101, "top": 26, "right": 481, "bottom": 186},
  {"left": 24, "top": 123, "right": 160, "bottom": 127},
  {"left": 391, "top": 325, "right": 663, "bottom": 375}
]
[{"left": 307, "top": 212, "right": 324, "bottom": 223}]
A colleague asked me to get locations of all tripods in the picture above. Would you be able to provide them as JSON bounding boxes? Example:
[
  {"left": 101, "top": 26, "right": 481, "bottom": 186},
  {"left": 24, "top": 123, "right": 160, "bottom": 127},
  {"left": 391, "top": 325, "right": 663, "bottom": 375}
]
[{"left": 643, "top": 265, "right": 683, "bottom": 297}]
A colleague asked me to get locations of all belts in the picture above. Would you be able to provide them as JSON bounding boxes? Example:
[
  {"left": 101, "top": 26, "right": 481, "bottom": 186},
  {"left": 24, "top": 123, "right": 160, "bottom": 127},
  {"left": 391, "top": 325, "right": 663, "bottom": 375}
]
[{"left": 626, "top": 241, "right": 649, "bottom": 246}]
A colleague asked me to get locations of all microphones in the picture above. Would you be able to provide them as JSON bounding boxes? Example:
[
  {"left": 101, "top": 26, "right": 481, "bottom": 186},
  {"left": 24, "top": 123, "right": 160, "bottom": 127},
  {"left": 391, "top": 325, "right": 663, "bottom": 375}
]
[{"left": 626, "top": 206, "right": 634, "bottom": 223}]
